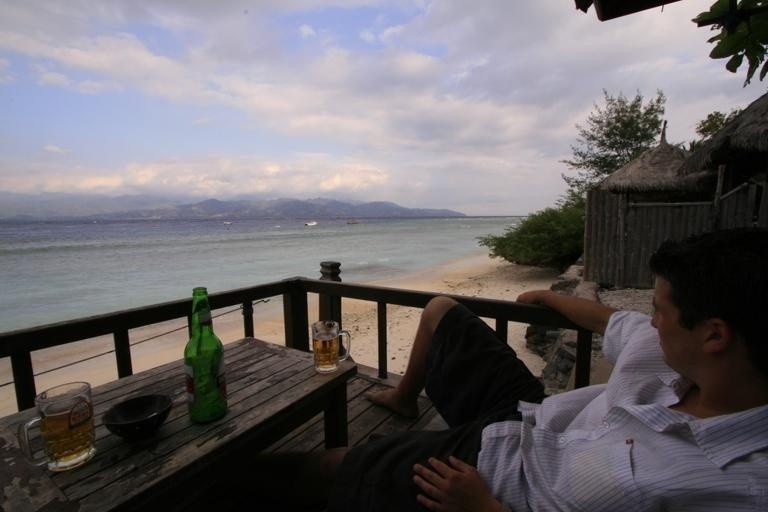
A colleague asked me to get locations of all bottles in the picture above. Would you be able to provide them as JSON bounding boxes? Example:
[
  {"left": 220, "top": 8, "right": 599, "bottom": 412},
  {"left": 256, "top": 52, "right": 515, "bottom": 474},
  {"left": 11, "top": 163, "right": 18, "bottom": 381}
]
[{"left": 183, "top": 286, "right": 228, "bottom": 423}]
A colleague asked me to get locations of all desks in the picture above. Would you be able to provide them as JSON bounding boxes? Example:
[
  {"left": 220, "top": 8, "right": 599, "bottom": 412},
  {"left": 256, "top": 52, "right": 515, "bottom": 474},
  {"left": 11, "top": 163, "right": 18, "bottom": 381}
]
[{"left": 0, "top": 337, "right": 357, "bottom": 512}]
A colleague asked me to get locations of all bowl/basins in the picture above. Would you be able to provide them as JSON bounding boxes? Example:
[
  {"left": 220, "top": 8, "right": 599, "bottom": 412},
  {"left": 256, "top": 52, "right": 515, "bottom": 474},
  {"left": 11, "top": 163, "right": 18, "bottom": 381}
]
[{"left": 102, "top": 392, "right": 173, "bottom": 437}]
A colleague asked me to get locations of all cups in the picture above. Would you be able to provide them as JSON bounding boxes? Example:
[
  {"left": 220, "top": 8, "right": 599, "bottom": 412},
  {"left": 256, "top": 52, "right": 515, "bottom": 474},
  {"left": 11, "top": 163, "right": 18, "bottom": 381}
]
[
  {"left": 18, "top": 380, "right": 97, "bottom": 474},
  {"left": 312, "top": 320, "right": 350, "bottom": 374}
]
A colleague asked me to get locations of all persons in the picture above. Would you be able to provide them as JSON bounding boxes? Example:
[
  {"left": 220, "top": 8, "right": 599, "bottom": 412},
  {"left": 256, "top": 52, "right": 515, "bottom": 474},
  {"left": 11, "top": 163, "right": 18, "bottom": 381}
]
[{"left": 239, "top": 223, "right": 767, "bottom": 509}]
